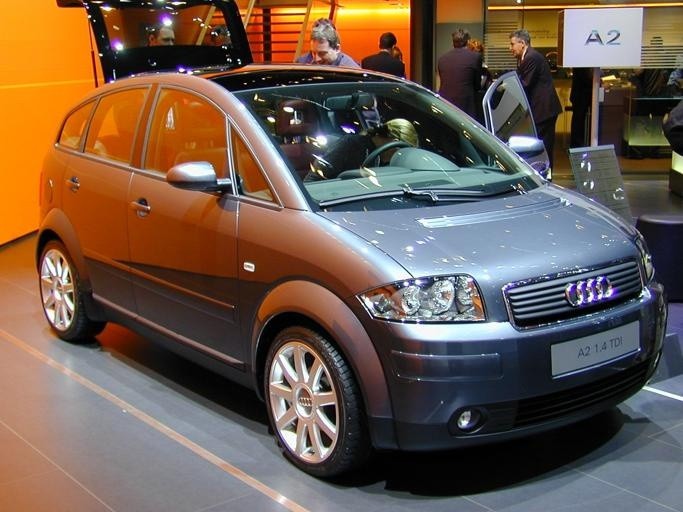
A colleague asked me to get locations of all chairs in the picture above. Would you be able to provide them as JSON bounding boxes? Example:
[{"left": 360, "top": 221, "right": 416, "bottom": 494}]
[
  {"left": 277, "top": 101, "right": 328, "bottom": 181},
  {"left": 173, "top": 103, "right": 228, "bottom": 180}
]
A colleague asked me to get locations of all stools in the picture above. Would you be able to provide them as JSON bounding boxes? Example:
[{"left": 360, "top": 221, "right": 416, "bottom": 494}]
[{"left": 636, "top": 215, "right": 683, "bottom": 303}]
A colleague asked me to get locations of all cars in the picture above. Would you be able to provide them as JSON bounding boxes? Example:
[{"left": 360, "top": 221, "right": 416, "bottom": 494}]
[{"left": 35, "top": 0, "right": 668, "bottom": 478}]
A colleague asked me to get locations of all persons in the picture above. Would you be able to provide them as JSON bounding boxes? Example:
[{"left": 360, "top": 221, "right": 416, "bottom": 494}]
[
  {"left": 665, "top": 54, "right": 682, "bottom": 99},
  {"left": 490, "top": 28, "right": 563, "bottom": 182},
  {"left": 144, "top": 22, "right": 177, "bottom": 47},
  {"left": 632, "top": 36, "right": 679, "bottom": 98},
  {"left": 293, "top": 23, "right": 381, "bottom": 130},
  {"left": 210, "top": 24, "right": 231, "bottom": 45},
  {"left": 437, "top": 28, "right": 484, "bottom": 121},
  {"left": 568, "top": 67, "right": 620, "bottom": 150},
  {"left": 302, "top": 117, "right": 419, "bottom": 184},
  {"left": 465, "top": 39, "right": 493, "bottom": 90},
  {"left": 294, "top": 17, "right": 337, "bottom": 64},
  {"left": 390, "top": 46, "right": 402, "bottom": 63},
  {"left": 361, "top": 32, "right": 405, "bottom": 77}
]
